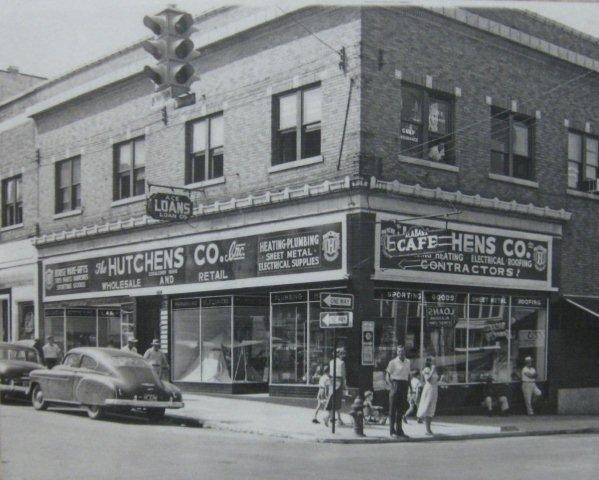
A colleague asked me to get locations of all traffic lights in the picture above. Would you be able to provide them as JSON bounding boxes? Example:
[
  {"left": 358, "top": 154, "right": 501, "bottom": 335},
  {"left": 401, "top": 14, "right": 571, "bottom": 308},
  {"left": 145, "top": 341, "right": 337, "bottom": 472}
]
[
  {"left": 140, "top": 11, "right": 168, "bottom": 90},
  {"left": 167, "top": 11, "right": 196, "bottom": 90}
]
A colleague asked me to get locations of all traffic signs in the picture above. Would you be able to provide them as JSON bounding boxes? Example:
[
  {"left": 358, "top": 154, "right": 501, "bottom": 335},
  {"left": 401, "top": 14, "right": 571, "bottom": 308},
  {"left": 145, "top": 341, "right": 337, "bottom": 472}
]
[
  {"left": 320, "top": 292, "right": 354, "bottom": 311},
  {"left": 319, "top": 311, "right": 353, "bottom": 329}
]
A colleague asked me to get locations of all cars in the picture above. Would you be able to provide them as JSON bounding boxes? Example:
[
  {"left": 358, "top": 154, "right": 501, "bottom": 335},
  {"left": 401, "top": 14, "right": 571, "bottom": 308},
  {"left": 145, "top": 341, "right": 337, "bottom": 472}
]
[
  {"left": 25, "top": 346, "right": 186, "bottom": 421},
  {"left": 0, "top": 341, "right": 49, "bottom": 402}
]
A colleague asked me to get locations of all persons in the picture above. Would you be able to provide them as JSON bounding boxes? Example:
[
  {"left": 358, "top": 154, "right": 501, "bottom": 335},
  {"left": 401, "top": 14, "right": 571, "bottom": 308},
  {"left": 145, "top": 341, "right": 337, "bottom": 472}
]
[
  {"left": 312, "top": 366, "right": 338, "bottom": 424},
  {"left": 142, "top": 338, "right": 170, "bottom": 379},
  {"left": 121, "top": 336, "right": 138, "bottom": 353},
  {"left": 32, "top": 338, "right": 43, "bottom": 358},
  {"left": 520, "top": 357, "right": 542, "bottom": 415},
  {"left": 481, "top": 376, "right": 509, "bottom": 417},
  {"left": 362, "top": 345, "right": 442, "bottom": 439},
  {"left": 41, "top": 336, "right": 62, "bottom": 370},
  {"left": 323, "top": 347, "right": 347, "bottom": 427},
  {"left": 428, "top": 140, "right": 446, "bottom": 162}
]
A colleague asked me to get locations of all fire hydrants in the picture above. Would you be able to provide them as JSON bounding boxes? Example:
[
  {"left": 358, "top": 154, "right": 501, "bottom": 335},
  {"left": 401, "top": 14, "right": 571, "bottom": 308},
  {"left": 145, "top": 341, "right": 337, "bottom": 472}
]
[{"left": 347, "top": 394, "right": 366, "bottom": 437}]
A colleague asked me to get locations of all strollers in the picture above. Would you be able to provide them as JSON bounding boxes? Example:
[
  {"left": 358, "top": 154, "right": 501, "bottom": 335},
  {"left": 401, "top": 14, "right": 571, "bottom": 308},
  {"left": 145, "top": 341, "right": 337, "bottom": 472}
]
[{"left": 345, "top": 387, "right": 387, "bottom": 426}]
